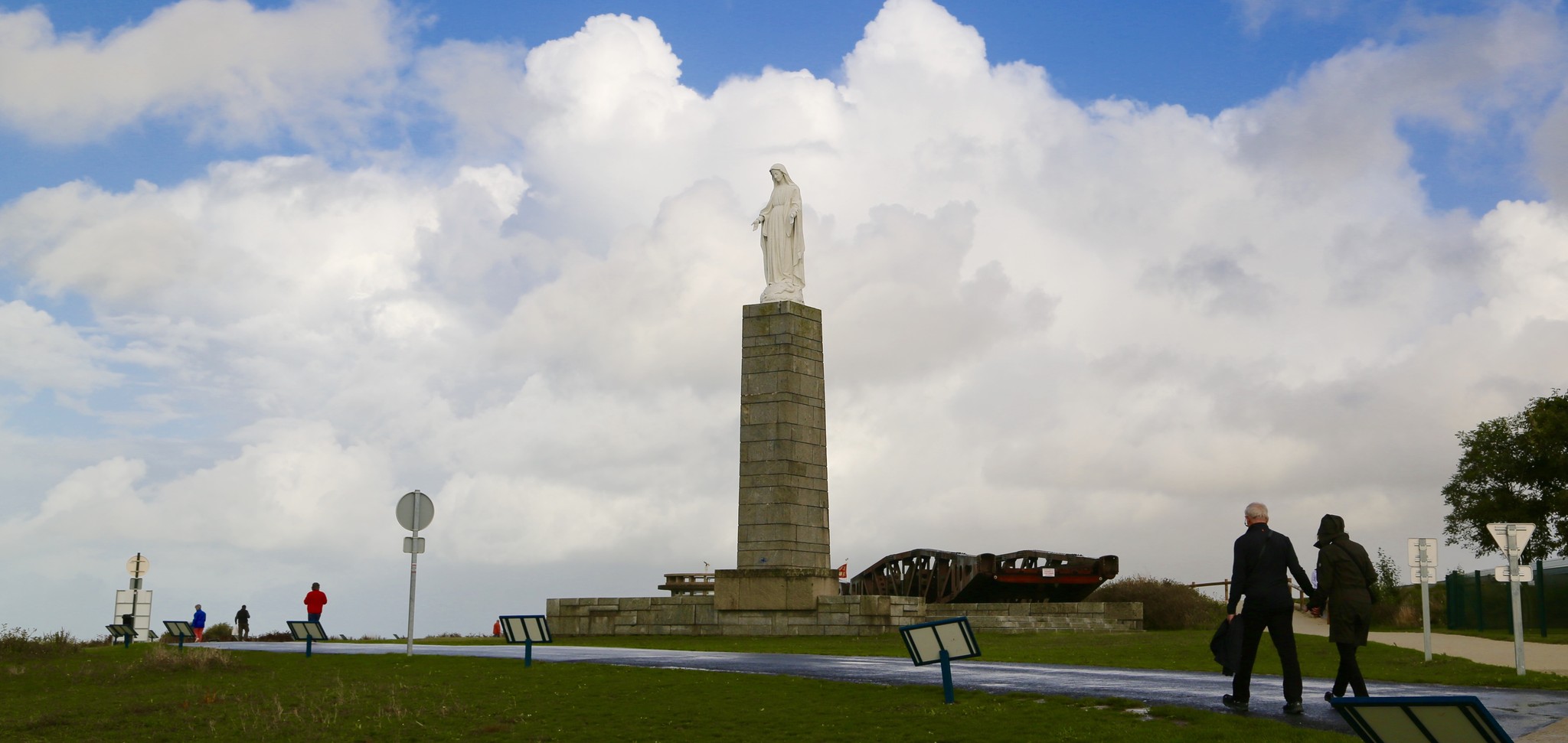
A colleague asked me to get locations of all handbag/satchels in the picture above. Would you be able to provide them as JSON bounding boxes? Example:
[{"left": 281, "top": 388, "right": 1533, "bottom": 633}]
[{"left": 1369, "top": 586, "right": 1379, "bottom": 604}]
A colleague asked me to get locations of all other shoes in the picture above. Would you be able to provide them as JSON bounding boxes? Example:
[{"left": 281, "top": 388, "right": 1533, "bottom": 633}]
[{"left": 195, "top": 638, "right": 199, "bottom": 643}]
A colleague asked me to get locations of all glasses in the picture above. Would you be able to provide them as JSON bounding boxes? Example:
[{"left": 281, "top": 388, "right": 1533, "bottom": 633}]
[{"left": 1245, "top": 518, "right": 1249, "bottom": 527}]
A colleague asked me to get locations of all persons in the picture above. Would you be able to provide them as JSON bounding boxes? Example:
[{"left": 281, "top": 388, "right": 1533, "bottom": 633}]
[
  {"left": 190, "top": 604, "right": 206, "bottom": 643},
  {"left": 304, "top": 583, "right": 327, "bottom": 642},
  {"left": 1304, "top": 513, "right": 1379, "bottom": 703},
  {"left": 235, "top": 605, "right": 250, "bottom": 641},
  {"left": 1223, "top": 502, "right": 1322, "bottom": 713},
  {"left": 492, "top": 620, "right": 500, "bottom": 637}
]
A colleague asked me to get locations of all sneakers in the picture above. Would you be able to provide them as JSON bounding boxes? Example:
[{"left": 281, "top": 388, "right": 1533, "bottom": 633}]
[
  {"left": 1223, "top": 694, "right": 1249, "bottom": 711},
  {"left": 1283, "top": 701, "right": 1304, "bottom": 716},
  {"left": 1325, "top": 692, "right": 1334, "bottom": 703}
]
